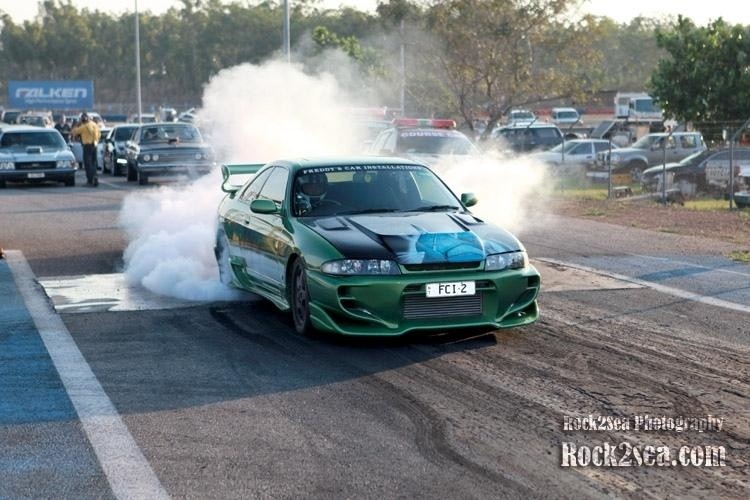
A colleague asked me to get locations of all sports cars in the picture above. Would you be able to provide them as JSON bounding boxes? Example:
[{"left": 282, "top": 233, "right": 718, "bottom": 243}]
[{"left": 214, "top": 156, "right": 542, "bottom": 341}]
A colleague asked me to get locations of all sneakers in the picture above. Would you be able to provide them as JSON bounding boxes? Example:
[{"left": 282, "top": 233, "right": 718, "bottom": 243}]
[
  {"left": 82, "top": 182, "right": 93, "bottom": 187},
  {"left": 93, "top": 181, "right": 99, "bottom": 187}
]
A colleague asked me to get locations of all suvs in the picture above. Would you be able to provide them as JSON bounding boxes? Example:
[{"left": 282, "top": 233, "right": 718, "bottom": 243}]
[
  {"left": 491, "top": 123, "right": 564, "bottom": 156},
  {"left": 597, "top": 132, "right": 707, "bottom": 183}
]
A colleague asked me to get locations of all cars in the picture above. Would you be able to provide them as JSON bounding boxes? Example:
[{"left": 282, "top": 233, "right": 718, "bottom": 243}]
[
  {"left": 369, "top": 119, "right": 487, "bottom": 168},
  {"left": 640, "top": 147, "right": 750, "bottom": 201},
  {"left": 0, "top": 109, "right": 217, "bottom": 187},
  {"left": 459, "top": 117, "right": 503, "bottom": 136},
  {"left": 509, "top": 111, "right": 537, "bottom": 124},
  {"left": 533, "top": 139, "right": 616, "bottom": 174},
  {"left": 734, "top": 165, "right": 750, "bottom": 207}
]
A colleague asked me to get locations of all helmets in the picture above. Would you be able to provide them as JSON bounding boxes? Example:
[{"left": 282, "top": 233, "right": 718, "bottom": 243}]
[{"left": 296, "top": 169, "right": 330, "bottom": 203}]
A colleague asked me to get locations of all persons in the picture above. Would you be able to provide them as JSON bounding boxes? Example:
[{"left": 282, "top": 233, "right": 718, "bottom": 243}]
[
  {"left": 53, "top": 112, "right": 101, "bottom": 186},
  {"left": 147, "top": 105, "right": 179, "bottom": 144},
  {"left": 363, "top": 176, "right": 402, "bottom": 210}
]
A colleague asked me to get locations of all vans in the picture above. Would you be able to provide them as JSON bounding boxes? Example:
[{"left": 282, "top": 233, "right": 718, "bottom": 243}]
[
  {"left": 627, "top": 97, "right": 663, "bottom": 119},
  {"left": 551, "top": 107, "right": 583, "bottom": 125}
]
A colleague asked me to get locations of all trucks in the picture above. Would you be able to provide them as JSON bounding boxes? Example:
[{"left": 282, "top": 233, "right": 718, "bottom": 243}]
[{"left": 615, "top": 93, "right": 629, "bottom": 118}]
